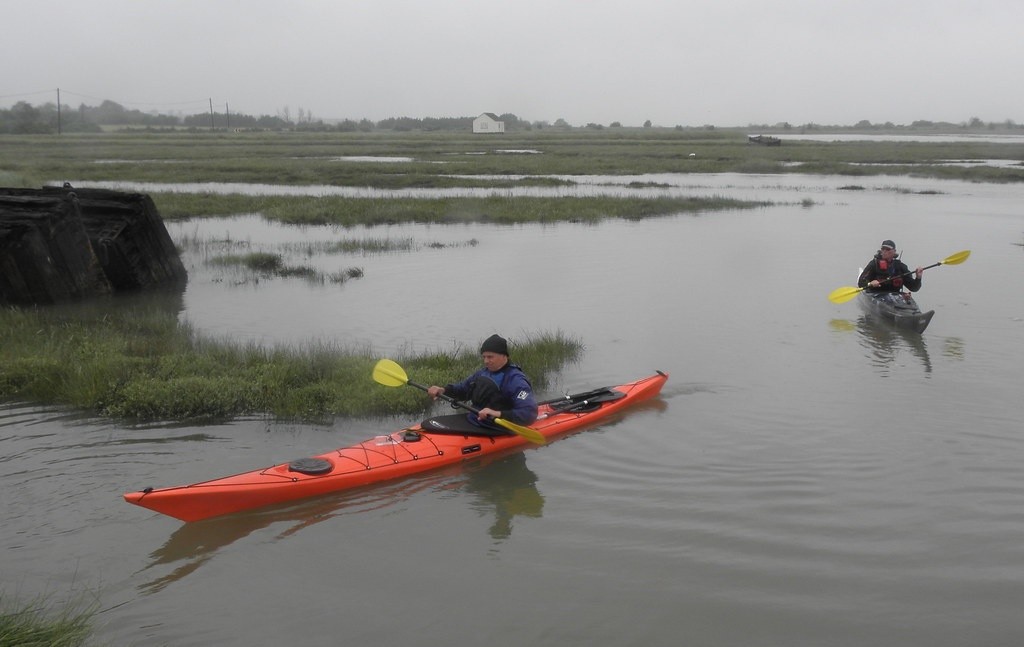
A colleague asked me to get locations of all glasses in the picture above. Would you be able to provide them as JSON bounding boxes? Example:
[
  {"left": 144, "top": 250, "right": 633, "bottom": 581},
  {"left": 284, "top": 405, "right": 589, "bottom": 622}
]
[{"left": 881, "top": 247, "right": 890, "bottom": 251}]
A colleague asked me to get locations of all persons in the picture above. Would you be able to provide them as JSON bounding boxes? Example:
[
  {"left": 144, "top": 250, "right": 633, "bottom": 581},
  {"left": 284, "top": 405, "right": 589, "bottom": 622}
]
[
  {"left": 428, "top": 334, "right": 538, "bottom": 432},
  {"left": 858, "top": 240, "right": 923, "bottom": 293}
]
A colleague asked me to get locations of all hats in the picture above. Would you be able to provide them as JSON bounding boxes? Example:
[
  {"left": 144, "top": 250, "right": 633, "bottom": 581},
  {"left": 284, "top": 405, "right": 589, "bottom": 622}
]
[
  {"left": 882, "top": 240, "right": 895, "bottom": 250},
  {"left": 480, "top": 334, "right": 509, "bottom": 357}
]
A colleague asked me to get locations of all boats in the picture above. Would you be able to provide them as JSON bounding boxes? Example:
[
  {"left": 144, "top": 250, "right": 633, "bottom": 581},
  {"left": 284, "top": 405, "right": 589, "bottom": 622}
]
[
  {"left": 124, "top": 372, "right": 670, "bottom": 523},
  {"left": 856, "top": 268, "right": 935, "bottom": 335}
]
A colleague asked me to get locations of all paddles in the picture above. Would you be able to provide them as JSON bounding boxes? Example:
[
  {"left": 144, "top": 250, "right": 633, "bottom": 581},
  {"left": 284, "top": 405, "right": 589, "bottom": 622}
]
[
  {"left": 829, "top": 250, "right": 971, "bottom": 304},
  {"left": 373, "top": 359, "right": 545, "bottom": 444}
]
